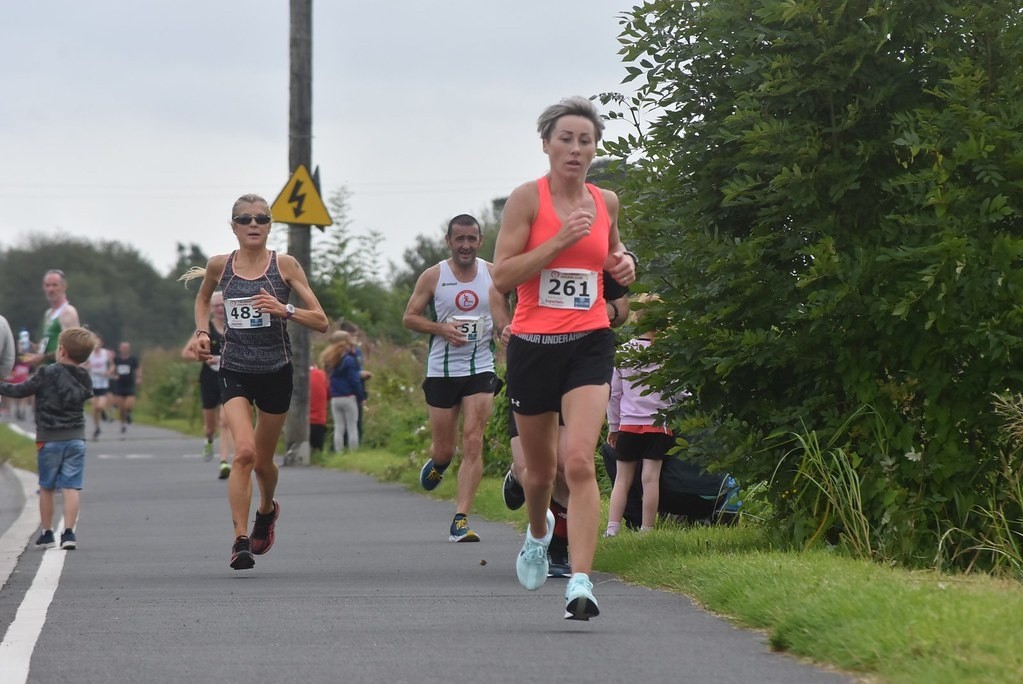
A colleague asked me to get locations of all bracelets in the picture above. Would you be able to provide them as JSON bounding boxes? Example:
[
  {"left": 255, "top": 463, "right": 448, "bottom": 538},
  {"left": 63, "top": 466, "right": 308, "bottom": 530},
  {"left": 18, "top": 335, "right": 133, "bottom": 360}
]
[
  {"left": 196, "top": 331, "right": 211, "bottom": 338},
  {"left": 608, "top": 302, "right": 619, "bottom": 322},
  {"left": 624, "top": 251, "right": 639, "bottom": 266},
  {"left": 285, "top": 304, "right": 295, "bottom": 318}
]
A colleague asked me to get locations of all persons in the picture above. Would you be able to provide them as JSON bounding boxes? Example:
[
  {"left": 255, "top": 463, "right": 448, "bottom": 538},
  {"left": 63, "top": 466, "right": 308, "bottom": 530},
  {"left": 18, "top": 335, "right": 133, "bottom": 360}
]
[
  {"left": 183, "top": 291, "right": 231, "bottom": 479},
  {"left": 602, "top": 294, "right": 693, "bottom": 538},
  {"left": 309, "top": 323, "right": 372, "bottom": 455},
  {"left": 402, "top": 215, "right": 511, "bottom": 543},
  {"left": 0, "top": 270, "right": 142, "bottom": 550},
  {"left": 491, "top": 97, "right": 637, "bottom": 621}
]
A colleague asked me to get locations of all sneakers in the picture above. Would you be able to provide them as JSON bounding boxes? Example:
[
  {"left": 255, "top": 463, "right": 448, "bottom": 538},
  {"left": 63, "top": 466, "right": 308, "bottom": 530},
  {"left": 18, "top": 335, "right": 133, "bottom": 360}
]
[
  {"left": 564, "top": 574, "right": 600, "bottom": 621},
  {"left": 502, "top": 470, "right": 526, "bottom": 510},
  {"left": 420, "top": 446, "right": 458, "bottom": 491},
  {"left": 546, "top": 533, "right": 572, "bottom": 578},
  {"left": 34, "top": 529, "right": 56, "bottom": 550},
  {"left": 59, "top": 528, "right": 77, "bottom": 550},
  {"left": 516, "top": 508, "right": 555, "bottom": 591},
  {"left": 218, "top": 463, "right": 231, "bottom": 479},
  {"left": 449, "top": 515, "right": 480, "bottom": 543},
  {"left": 249, "top": 498, "right": 280, "bottom": 555},
  {"left": 230, "top": 535, "right": 255, "bottom": 570},
  {"left": 203, "top": 439, "right": 214, "bottom": 462}
]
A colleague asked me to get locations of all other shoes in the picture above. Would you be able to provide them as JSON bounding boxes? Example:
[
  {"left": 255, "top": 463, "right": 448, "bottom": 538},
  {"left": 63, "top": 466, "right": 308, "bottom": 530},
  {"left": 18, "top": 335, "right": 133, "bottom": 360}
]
[
  {"left": 126, "top": 414, "right": 132, "bottom": 424},
  {"left": 93, "top": 427, "right": 100, "bottom": 437},
  {"left": 101, "top": 411, "right": 106, "bottom": 421},
  {"left": 121, "top": 426, "right": 126, "bottom": 433}
]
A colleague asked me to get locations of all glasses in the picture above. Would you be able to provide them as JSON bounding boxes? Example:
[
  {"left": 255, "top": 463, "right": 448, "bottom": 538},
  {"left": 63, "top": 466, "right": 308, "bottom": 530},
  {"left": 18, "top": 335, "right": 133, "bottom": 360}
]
[{"left": 232, "top": 214, "right": 271, "bottom": 225}]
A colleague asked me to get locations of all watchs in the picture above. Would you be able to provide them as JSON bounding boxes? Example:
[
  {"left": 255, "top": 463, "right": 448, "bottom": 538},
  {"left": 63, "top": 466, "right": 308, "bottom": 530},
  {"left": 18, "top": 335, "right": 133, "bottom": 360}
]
[{"left": 192, "top": 193, "right": 329, "bottom": 570}]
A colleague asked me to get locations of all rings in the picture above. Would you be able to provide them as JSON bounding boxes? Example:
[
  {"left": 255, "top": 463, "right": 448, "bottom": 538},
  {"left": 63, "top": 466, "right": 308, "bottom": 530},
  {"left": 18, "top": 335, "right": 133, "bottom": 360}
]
[
  {"left": 621, "top": 277, "right": 627, "bottom": 282},
  {"left": 617, "top": 273, "right": 621, "bottom": 278}
]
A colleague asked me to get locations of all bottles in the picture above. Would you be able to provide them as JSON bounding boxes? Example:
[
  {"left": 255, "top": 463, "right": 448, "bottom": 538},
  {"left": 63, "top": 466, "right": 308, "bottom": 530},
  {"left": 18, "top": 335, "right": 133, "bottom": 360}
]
[{"left": 20, "top": 331, "right": 29, "bottom": 351}]
